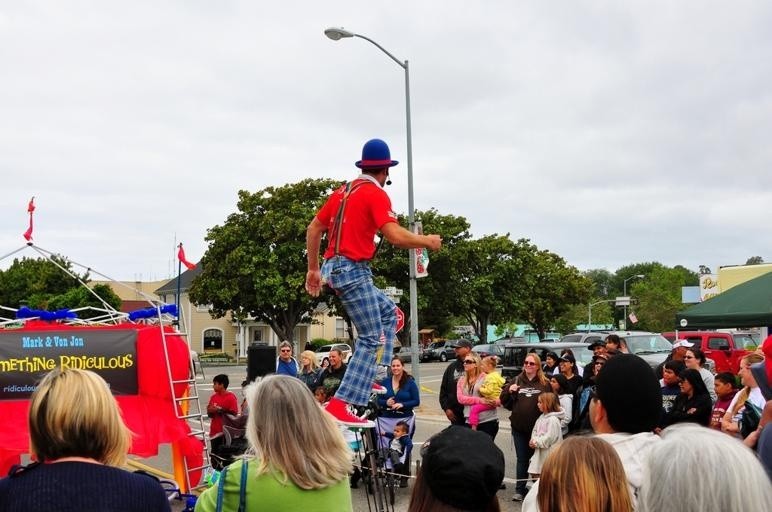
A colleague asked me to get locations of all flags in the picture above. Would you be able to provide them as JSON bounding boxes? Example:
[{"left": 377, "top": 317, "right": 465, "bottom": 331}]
[
  {"left": 23, "top": 200, "right": 37, "bottom": 241},
  {"left": 178, "top": 244, "right": 197, "bottom": 272}
]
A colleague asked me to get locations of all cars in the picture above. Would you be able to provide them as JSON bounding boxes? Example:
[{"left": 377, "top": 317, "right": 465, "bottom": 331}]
[
  {"left": 471, "top": 344, "right": 505, "bottom": 366},
  {"left": 188, "top": 349, "right": 200, "bottom": 381},
  {"left": 392, "top": 347, "right": 422, "bottom": 364},
  {"left": 315, "top": 343, "right": 352, "bottom": 368}
]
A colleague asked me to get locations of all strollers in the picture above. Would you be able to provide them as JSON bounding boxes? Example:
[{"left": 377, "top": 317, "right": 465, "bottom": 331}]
[{"left": 350, "top": 405, "right": 416, "bottom": 496}]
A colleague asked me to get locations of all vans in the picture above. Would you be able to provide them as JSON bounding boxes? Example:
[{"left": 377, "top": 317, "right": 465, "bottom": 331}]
[{"left": 659, "top": 331, "right": 759, "bottom": 376}]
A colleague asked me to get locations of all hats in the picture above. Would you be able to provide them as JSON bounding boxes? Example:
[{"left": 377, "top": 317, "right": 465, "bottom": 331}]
[
  {"left": 592, "top": 353, "right": 611, "bottom": 361},
  {"left": 355, "top": 139, "right": 399, "bottom": 169},
  {"left": 552, "top": 374, "right": 566, "bottom": 386},
  {"left": 588, "top": 340, "right": 606, "bottom": 350},
  {"left": 559, "top": 354, "right": 574, "bottom": 362},
  {"left": 672, "top": 338, "right": 695, "bottom": 350},
  {"left": 450, "top": 338, "right": 473, "bottom": 349}
]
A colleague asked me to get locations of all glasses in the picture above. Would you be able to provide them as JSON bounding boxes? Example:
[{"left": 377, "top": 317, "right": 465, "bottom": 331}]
[
  {"left": 524, "top": 361, "right": 536, "bottom": 366},
  {"left": 282, "top": 350, "right": 290, "bottom": 352},
  {"left": 547, "top": 352, "right": 554, "bottom": 355},
  {"left": 463, "top": 360, "right": 476, "bottom": 364},
  {"left": 683, "top": 355, "right": 695, "bottom": 359},
  {"left": 594, "top": 362, "right": 604, "bottom": 365}
]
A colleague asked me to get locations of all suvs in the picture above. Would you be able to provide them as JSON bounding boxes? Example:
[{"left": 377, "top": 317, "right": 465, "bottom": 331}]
[
  {"left": 582, "top": 330, "right": 718, "bottom": 380},
  {"left": 496, "top": 342, "right": 593, "bottom": 386},
  {"left": 423, "top": 341, "right": 457, "bottom": 363}
]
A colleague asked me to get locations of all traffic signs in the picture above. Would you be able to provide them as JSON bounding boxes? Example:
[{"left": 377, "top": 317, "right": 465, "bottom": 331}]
[{"left": 395, "top": 304, "right": 405, "bottom": 334}]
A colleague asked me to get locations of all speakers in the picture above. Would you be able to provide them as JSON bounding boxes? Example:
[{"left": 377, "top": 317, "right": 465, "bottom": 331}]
[{"left": 247, "top": 345, "right": 277, "bottom": 380}]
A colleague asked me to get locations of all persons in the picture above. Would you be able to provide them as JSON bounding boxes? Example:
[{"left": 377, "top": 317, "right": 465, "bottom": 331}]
[
  {"left": 375, "top": 356, "right": 420, "bottom": 411},
  {"left": 646, "top": 424, "right": 772, "bottom": 511},
  {"left": 377, "top": 422, "right": 412, "bottom": 474},
  {"left": 560, "top": 349, "right": 573, "bottom": 358},
  {"left": 0, "top": 367, "right": 170, "bottom": 512},
  {"left": 195, "top": 375, "right": 354, "bottom": 512},
  {"left": 606, "top": 335, "right": 620, "bottom": 355},
  {"left": 723, "top": 354, "right": 765, "bottom": 437},
  {"left": 314, "top": 386, "right": 330, "bottom": 404},
  {"left": 655, "top": 339, "right": 696, "bottom": 378},
  {"left": 671, "top": 369, "right": 712, "bottom": 425},
  {"left": 658, "top": 360, "right": 685, "bottom": 413},
  {"left": 500, "top": 353, "right": 556, "bottom": 502},
  {"left": 204, "top": 375, "right": 239, "bottom": 449},
  {"left": 557, "top": 355, "right": 582, "bottom": 397},
  {"left": 534, "top": 437, "right": 634, "bottom": 512},
  {"left": 522, "top": 353, "right": 661, "bottom": 512},
  {"left": 439, "top": 339, "right": 474, "bottom": 424},
  {"left": 683, "top": 349, "right": 718, "bottom": 403},
  {"left": 618, "top": 338, "right": 631, "bottom": 354},
  {"left": 543, "top": 353, "right": 560, "bottom": 375},
  {"left": 305, "top": 140, "right": 442, "bottom": 426},
  {"left": 318, "top": 348, "right": 348, "bottom": 392},
  {"left": 550, "top": 375, "right": 575, "bottom": 437},
  {"left": 468, "top": 355, "right": 505, "bottom": 431},
  {"left": 275, "top": 341, "right": 300, "bottom": 376},
  {"left": 296, "top": 351, "right": 323, "bottom": 388},
  {"left": 581, "top": 339, "right": 606, "bottom": 386},
  {"left": 589, "top": 354, "right": 608, "bottom": 374},
  {"left": 710, "top": 372, "right": 739, "bottom": 429},
  {"left": 528, "top": 392, "right": 566, "bottom": 475}
]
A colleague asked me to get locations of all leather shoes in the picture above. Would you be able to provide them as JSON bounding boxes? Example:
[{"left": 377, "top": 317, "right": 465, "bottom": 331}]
[{"left": 499, "top": 483, "right": 506, "bottom": 489}]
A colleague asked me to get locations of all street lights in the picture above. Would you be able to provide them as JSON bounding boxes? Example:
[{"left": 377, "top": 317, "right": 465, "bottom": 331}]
[
  {"left": 624, "top": 275, "right": 644, "bottom": 330},
  {"left": 325, "top": 27, "right": 419, "bottom": 388}
]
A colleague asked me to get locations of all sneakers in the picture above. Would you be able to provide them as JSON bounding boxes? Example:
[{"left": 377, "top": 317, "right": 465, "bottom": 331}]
[
  {"left": 324, "top": 396, "right": 375, "bottom": 430},
  {"left": 372, "top": 384, "right": 387, "bottom": 395},
  {"left": 400, "top": 479, "right": 408, "bottom": 488},
  {"left": 513, "top": 493, "right": 523, "bottom": 500}
]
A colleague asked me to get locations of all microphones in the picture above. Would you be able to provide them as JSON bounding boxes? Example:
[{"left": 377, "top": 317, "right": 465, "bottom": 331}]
[{"left": 386, "top": 179, "right": 392, "bottom": 186}]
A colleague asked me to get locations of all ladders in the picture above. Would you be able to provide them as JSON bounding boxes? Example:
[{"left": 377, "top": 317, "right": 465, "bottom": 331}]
[{"left": 154, "top": 304, "right": 213, "bottom": 497}]
